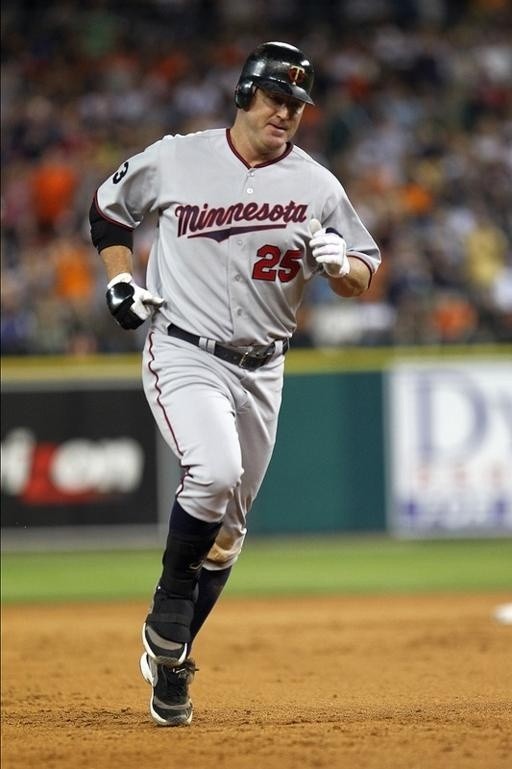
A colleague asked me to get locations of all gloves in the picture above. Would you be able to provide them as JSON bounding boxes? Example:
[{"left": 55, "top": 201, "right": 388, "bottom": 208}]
[
  {"left": 308, "top": 218, "right": 350, "bottom": 279},
  {"left": 106, "top": 272, "right": 167, "bottom": 330}
]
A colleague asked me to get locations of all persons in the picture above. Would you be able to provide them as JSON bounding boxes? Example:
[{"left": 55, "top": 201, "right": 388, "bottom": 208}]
[
  {"left": 88, "top": 39, "right": 386, "bottom": 728},
  {"left": 0, "top": 0, "right": 512, "bottom": 359}
]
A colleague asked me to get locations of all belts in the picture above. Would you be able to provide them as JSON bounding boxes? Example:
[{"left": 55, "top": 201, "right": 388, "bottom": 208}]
[{"left": 167, "top": 320, "right": 289, "bottom": 370}]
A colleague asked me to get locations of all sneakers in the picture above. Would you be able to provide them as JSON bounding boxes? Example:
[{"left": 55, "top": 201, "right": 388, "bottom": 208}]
[
  {"left": 141, "top": 574, "right": 200, "bottom": 668},
  {"left": 139, "top": 649, "right": 200, "bottom": 727}
]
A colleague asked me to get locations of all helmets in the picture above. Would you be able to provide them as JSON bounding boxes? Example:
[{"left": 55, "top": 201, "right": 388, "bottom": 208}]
[{"left": 233, "top": 41, "right": 317, "bottom": 109}]
